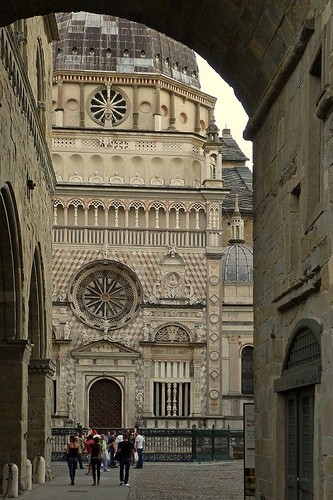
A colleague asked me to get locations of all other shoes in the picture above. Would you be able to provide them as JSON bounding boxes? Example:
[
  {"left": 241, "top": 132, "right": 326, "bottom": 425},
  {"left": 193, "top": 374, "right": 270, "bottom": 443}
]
[
  {"left": 118, "top": 481, "right": 123, "bottom": 485},
  {"left": 124, "top": 484, "right": 129, "bottom": 486}
]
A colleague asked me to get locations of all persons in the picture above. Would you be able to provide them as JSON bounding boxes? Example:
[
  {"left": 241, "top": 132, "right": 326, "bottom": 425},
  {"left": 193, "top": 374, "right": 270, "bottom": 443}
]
[
  {"left": 73, "top": 436, "right": 85, "bottom": 469},
  {"left": 65, "top": 435, "right": 80, "bottom": 486},
  {"left": 78, "top": 437, "right": 102, "bottom": 486},
  {"left": 82, "top": 435, "right": 95, "bottom": 475},
  {"left": 116, "top": 434, "right": 135, "bottom": 486},
  {"left": 84, "top": 426, "right": 145, "bottom": 472}
]
